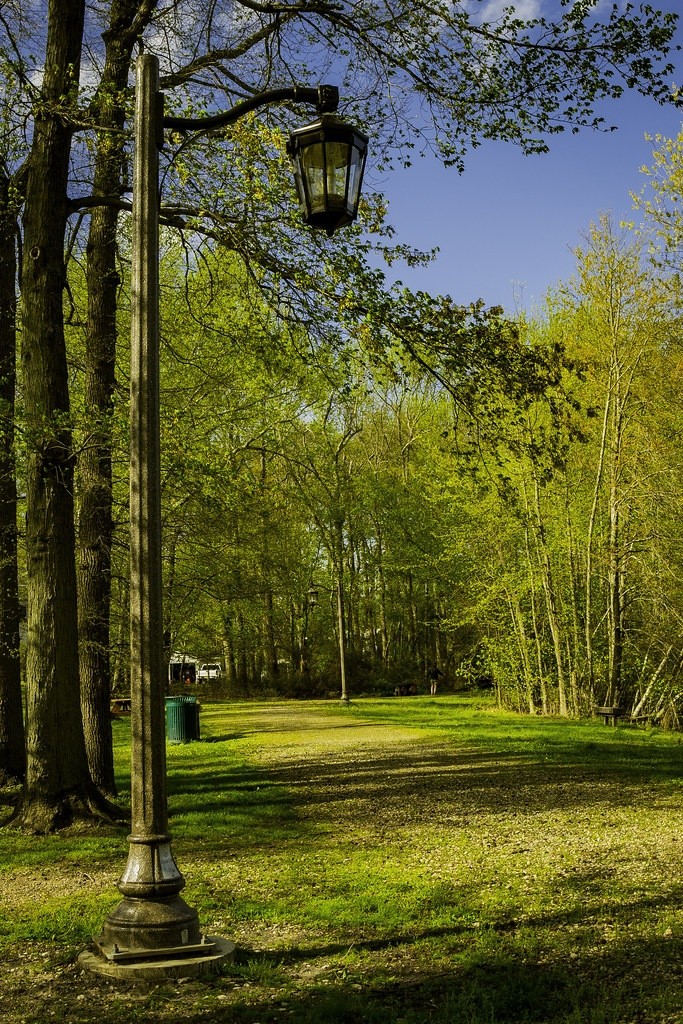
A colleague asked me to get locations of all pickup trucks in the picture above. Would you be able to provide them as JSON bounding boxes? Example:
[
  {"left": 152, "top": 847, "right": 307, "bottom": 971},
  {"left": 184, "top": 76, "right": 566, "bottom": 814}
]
[{"left": 197, "top": 664, "right": 223, "bottom": 684}]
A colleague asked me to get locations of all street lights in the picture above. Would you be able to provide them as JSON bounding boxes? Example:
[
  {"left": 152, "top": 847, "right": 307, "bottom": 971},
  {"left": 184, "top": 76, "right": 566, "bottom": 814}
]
[{"left": 74, "top": 51, "right": 370, "bottom": 979}]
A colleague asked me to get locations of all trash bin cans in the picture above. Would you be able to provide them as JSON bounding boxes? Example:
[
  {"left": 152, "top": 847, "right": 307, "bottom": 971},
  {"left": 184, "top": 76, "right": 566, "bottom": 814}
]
[{"left": 165, "top": 696, "right": 200, "bottom": 743}]
